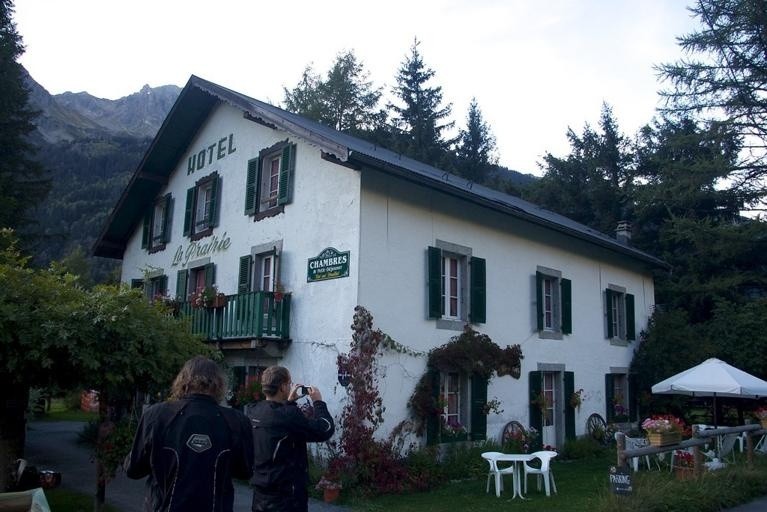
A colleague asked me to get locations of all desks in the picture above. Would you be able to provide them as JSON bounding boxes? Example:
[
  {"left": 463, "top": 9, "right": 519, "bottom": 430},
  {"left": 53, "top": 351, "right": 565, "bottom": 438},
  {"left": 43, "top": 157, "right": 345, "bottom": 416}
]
[{"left": 493, "top": 454, "right": 537, "bottom": 503}]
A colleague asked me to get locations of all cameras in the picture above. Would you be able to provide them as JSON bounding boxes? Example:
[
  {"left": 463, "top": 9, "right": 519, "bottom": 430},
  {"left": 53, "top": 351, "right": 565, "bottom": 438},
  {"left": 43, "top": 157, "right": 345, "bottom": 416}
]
[{"left": 302, "top": 387, "right": 311, "bottom": 395}]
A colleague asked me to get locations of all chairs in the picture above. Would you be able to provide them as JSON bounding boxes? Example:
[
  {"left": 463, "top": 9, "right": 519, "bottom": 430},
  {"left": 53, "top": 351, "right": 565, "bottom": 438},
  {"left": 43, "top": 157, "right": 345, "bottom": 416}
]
[
  {"left": 481, "top": 450, "right": 558, "bottom": 497},
  {"left": 736, "top": 431, "right": 746, "bottom": 452},
  {"left": 614, "top": 432, "right": 661, "bottom": 472}
]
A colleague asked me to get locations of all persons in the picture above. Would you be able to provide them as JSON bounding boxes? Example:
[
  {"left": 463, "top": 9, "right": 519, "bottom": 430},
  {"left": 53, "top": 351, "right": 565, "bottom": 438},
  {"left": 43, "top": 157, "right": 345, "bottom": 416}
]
[
  {"left": 120, "top": 355, "right": 254, "bottom": 511},
  {"left": 240, "top": 363, "right": 334, "bottom": 511}
]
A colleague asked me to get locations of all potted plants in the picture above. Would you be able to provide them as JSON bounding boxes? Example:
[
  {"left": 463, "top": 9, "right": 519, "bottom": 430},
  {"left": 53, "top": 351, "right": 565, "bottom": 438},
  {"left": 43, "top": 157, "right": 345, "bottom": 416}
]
[{"left": 273, "top": 285, "right": 284, "bottom": 303}]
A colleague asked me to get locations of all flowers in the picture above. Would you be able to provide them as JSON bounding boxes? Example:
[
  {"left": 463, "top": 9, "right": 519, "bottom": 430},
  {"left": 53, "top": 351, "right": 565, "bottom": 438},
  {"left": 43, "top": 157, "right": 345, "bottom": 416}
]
[
  {"left": 191, "top": 284, "right": 219, "bottom": 308},
  {"left": 674, "top": 450, "right": 694, "bottom": 468},
  {"left": 314, "top": 475, "right": 342, "bottom": 491},
  {"left": 642, "top": 416, "right": 677, "bottom": 434}
]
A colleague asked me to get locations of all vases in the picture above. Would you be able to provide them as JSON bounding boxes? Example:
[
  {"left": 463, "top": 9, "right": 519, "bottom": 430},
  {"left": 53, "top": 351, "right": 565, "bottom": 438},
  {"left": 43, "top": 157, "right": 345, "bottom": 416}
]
[
  {"left": 323, "top": 489, "right": 340, "bottom": 503},
  {"left": 677, "top": 468, "right": 697, "bottom": 481},
  {"left": 205, "top": 293, "right": 225, "bottom": 310}
]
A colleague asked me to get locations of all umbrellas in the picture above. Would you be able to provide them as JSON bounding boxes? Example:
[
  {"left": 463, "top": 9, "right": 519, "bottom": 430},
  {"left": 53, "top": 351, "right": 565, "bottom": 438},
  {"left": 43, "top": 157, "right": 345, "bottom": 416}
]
[{"left": 649, "top": 356, "right": 766, "bottom": 461}]
